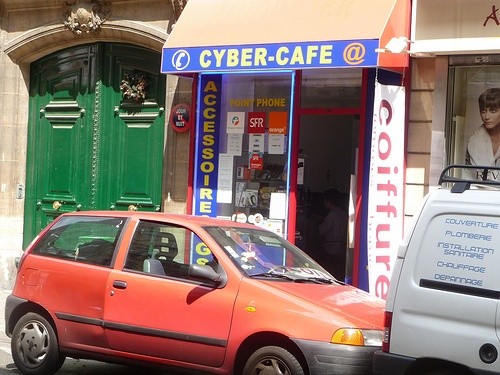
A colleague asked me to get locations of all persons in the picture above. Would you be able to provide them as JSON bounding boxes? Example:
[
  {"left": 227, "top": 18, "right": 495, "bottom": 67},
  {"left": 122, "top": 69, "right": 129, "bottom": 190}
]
[
  {"left": 310, "top": 188, "right": 347, "bottom": 280},
  {"left": 462, "top": 88, "right": 500, "bottom": 181}
]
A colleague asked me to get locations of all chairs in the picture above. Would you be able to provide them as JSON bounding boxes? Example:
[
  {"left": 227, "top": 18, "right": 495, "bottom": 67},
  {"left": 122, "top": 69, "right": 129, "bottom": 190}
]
[
  {"left": 143, "top": 232, "right": 182, "bottom": 277},
  {"left": 73, "top": 238, "right": 114, "bottom": 265}
]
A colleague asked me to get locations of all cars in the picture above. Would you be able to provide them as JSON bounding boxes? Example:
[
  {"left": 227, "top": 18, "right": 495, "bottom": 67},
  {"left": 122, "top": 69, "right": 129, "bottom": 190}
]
[{"left": 4, "top": 209, "right": 386, "bottom": 375}]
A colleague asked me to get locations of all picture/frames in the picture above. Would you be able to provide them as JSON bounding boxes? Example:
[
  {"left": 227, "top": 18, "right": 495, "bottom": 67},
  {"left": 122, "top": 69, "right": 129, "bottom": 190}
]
[{"left": 444, "top": 62, "right": 500, "bottom": 190}]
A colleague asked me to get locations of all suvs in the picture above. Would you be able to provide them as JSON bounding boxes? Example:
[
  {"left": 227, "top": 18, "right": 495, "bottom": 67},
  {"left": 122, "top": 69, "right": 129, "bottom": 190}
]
[{"left": 371, "top": 163, "right": 500, "bottom": 375}]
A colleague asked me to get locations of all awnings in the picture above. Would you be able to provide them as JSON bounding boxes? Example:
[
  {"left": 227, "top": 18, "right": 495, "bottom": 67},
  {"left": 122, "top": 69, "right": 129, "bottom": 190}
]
[{"left": 160, "top": 0, "right": 412, "bottom": 77}]
